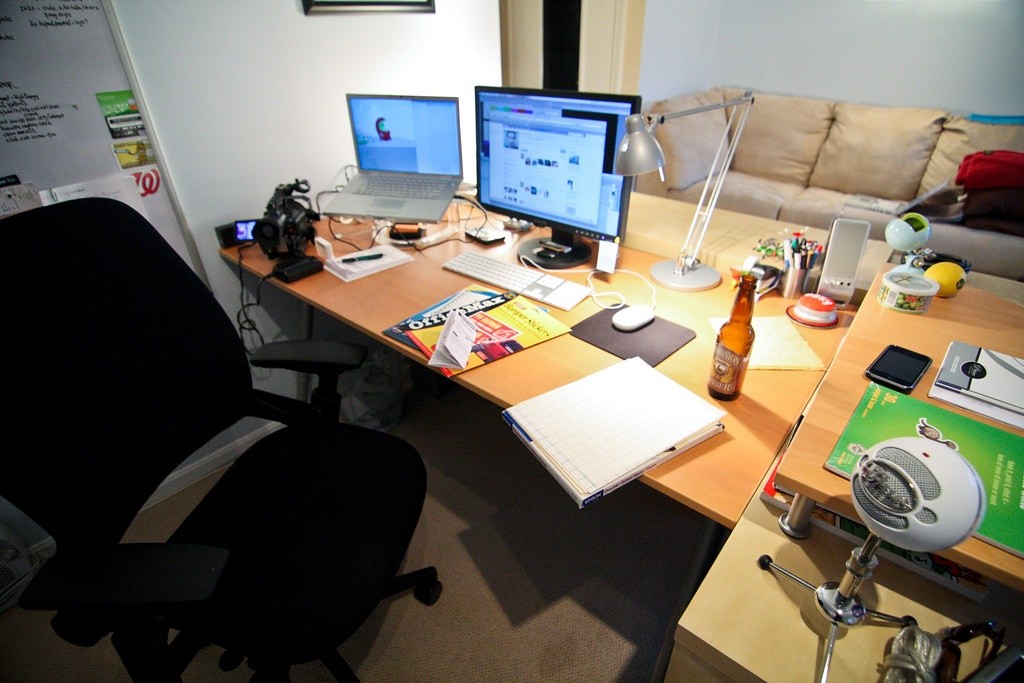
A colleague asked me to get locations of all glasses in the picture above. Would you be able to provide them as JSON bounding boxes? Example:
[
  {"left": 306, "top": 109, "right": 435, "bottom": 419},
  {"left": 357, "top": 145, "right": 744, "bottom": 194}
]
[{"left": 935, "top": 624, "right": 1006, "bottom": 683}]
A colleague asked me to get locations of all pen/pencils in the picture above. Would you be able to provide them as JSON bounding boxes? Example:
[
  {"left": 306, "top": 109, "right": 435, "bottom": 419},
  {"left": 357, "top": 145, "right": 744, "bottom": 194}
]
[{"left": 341, "top": 254, "right": 383, "bottom": 263}]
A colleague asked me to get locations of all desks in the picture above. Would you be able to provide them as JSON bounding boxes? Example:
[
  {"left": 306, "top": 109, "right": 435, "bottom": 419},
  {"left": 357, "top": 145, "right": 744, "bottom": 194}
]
[
  {"left": 666, "top": 262, "right": 1024, "bottom": 682},
  {"left": 221, "top": 195, "right": 857, "bottom": 526}
]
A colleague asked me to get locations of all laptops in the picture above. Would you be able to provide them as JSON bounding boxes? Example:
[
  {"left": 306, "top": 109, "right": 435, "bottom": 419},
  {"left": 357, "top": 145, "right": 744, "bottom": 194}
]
[{"left": 322, "top": 94, "right": 464, "bottom": 224}]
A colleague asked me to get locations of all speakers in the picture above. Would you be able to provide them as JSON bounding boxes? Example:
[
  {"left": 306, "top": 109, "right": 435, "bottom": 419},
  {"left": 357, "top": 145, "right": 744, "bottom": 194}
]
[{"left": 816, "top": 217, "right": 872, "bottom": 310}]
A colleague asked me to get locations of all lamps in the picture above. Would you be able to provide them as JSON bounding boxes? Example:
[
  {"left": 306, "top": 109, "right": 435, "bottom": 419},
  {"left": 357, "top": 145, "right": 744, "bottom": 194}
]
[{"left": 613, "top": 90, "right": 756, "bottom": 294}]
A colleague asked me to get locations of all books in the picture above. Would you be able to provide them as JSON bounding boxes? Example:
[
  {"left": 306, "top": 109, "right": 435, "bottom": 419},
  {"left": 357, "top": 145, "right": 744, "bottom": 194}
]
[
  {"left": 379, "top": 281, "right": 571, "bottom": 378},
  {"left": 824, "top": 381, "right": 1024, "bottom": 558},
  {"left": 927, "top": 340, "right": 1023, "bottom": 433},
  {"left": 501, "top": 356, "right": 725, "bottom": 509}
]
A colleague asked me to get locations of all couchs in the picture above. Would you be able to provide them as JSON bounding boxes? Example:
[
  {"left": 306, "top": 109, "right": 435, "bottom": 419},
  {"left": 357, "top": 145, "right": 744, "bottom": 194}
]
[{"left": 640, "top": 87, "right": 1024, "bottom": 308}]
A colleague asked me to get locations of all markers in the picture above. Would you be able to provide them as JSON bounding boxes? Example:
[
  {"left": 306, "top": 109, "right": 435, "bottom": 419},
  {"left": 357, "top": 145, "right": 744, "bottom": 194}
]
[{"left": 784, "top": 238, "right": 822, "bottom": 271}]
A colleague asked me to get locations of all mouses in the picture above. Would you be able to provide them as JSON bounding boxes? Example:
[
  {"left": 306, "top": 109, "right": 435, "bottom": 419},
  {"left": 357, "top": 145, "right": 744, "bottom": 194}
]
[{"left": 612, "top": 306, "right": 655, "bottom": 331}]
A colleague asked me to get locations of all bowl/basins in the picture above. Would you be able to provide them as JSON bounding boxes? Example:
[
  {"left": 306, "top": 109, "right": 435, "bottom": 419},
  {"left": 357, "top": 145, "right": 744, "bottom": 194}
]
[{"left": 877, "top": 271, "right": 940, "bottom": 314}]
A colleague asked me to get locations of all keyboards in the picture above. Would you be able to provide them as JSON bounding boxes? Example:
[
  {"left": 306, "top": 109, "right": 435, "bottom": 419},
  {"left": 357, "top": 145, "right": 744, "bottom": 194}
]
[{"left": 441, "top": 250, "right": 593, "bottom": 311}]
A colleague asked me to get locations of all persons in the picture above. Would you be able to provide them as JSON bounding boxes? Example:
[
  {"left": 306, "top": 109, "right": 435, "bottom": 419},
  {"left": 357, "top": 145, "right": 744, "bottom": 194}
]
[{"left": 505, "top": 131, "right": 519, "bottom": 150}]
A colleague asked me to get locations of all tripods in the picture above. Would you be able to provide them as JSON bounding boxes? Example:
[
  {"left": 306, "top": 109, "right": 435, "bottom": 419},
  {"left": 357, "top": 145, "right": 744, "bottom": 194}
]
[{"left": 759, "top": 531, "right": 918, "bottom": 683}]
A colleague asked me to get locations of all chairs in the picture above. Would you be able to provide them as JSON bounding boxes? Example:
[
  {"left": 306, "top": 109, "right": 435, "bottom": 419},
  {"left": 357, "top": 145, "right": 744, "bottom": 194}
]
[{"left": 0, "top": 198, "right": 443, "bottom": 683}]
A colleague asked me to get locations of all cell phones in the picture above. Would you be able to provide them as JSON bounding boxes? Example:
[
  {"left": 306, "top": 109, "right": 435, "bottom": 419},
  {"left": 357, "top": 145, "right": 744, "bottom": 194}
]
[{"left": 864, "top": 345, "right": 932, "bottom": 394}]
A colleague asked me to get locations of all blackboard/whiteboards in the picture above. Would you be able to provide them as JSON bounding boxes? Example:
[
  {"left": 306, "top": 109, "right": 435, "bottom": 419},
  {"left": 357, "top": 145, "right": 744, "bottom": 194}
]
[{"left": 0, "top": 0, "right": 211, "bottom": 291}]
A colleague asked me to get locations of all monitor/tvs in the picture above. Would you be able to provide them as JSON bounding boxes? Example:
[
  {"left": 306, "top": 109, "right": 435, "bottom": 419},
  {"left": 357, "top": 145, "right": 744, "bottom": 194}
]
[{"left": 474, "top": 85, "right": 642, "bottom": 270}]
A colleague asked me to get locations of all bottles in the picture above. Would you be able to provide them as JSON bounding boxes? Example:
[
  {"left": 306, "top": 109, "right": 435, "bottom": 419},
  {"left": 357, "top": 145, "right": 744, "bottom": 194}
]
[{"left": 707, "top": 275, "right": 759, "bottom": 403}]
